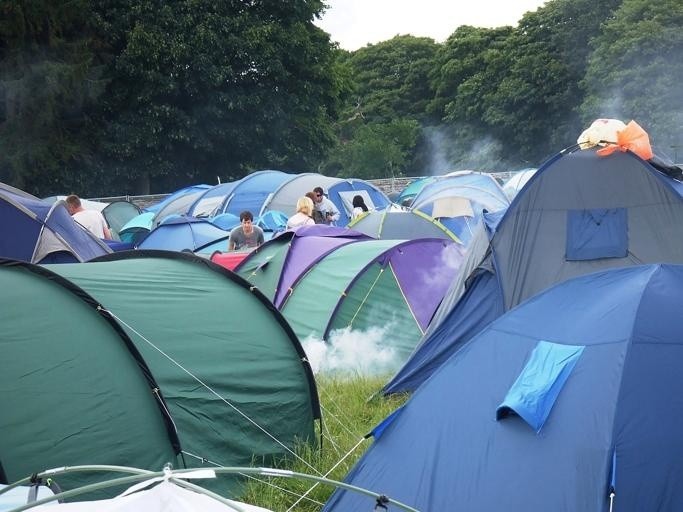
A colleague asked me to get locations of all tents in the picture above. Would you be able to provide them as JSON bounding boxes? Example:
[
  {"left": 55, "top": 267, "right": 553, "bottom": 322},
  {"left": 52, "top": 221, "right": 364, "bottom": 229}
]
[{"left": 1, "top": 119, "right": 683, "bottom": 512}]
